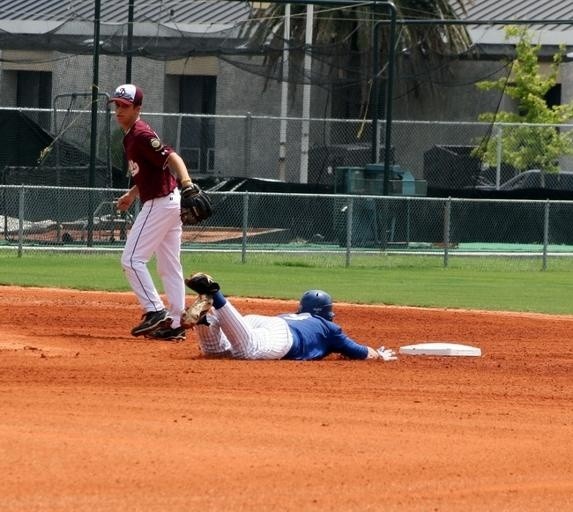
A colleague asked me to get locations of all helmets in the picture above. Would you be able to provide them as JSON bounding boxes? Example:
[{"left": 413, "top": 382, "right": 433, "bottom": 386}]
[{"left": 299, "top": 288, "right": 336, "bottom": 319}]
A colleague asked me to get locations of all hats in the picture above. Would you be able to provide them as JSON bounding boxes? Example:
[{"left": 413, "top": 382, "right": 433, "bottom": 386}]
[{"left": 106, "top": 83, "right": 144, "bottom": 107}]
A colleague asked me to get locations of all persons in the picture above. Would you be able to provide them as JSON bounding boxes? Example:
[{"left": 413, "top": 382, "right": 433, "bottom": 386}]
[
  {"left": 105, "top": 82, "right": 216, "bottom": 344},
  {"left": 181, "top": 270, "right": 403, "bottom": 363}
]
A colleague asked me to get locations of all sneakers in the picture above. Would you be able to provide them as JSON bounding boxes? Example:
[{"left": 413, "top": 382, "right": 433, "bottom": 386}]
[
  {"left": 179, "top": 293, "right": 214, "bottom": 330},
  {"left": 143, "top": 325, "right": 187, "bottom": 341},
  {"left": 129, "top": 307, "right": 175, "bottom": 337},
  {"left": 183, "top": 270, "right": 221, "bottom": 295}
]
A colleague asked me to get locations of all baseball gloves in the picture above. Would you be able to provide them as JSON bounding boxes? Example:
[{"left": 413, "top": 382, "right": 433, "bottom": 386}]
[{"left": 180, "top": 183, "right": 213, "bottom": 225}]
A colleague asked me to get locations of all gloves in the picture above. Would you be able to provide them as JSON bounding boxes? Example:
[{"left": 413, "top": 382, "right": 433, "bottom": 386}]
[{"left": 366, "top": 345, "right": 401, "bottom": 361}]
